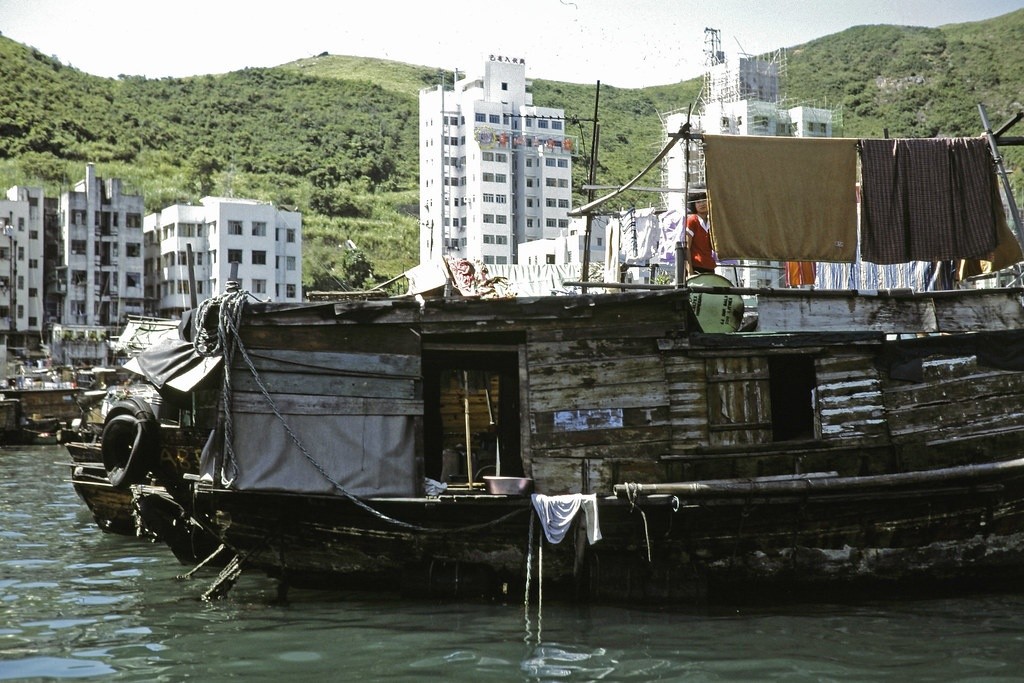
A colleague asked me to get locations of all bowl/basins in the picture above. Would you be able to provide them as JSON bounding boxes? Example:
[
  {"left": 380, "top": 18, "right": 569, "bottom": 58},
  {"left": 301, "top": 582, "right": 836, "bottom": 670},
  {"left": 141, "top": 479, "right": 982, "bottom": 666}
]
[{"left": 484, "top": 476, "right": 533, "bottom": 495}]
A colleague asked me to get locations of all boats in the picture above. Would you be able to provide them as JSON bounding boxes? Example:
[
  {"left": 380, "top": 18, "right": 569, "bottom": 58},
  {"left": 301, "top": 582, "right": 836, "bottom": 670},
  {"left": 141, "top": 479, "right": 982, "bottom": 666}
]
[
  {"left": 70, "top": 461, "right": 190, "bottom": 535},
  {"left": 65, "top": 442, "right": 106, "bottom": 461},
  {"left": 133, "top": 484, "right": 239, "bottom": 566},
  {"left": 100, "top": 286, "right": 1023, "bottom": 604}
]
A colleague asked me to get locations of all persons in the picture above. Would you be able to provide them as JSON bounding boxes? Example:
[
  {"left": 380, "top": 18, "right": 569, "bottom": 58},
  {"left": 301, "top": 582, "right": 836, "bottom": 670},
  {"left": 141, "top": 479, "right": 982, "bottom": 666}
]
[{"left": 685, "top": 192, "right": 716, "bottom": 275}]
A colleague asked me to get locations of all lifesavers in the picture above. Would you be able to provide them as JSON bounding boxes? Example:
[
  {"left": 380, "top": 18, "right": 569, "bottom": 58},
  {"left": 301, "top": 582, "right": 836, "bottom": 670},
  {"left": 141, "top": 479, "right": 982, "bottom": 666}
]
[{"left": 99, "top": 394, "right": 163, "bottom": 495}]
